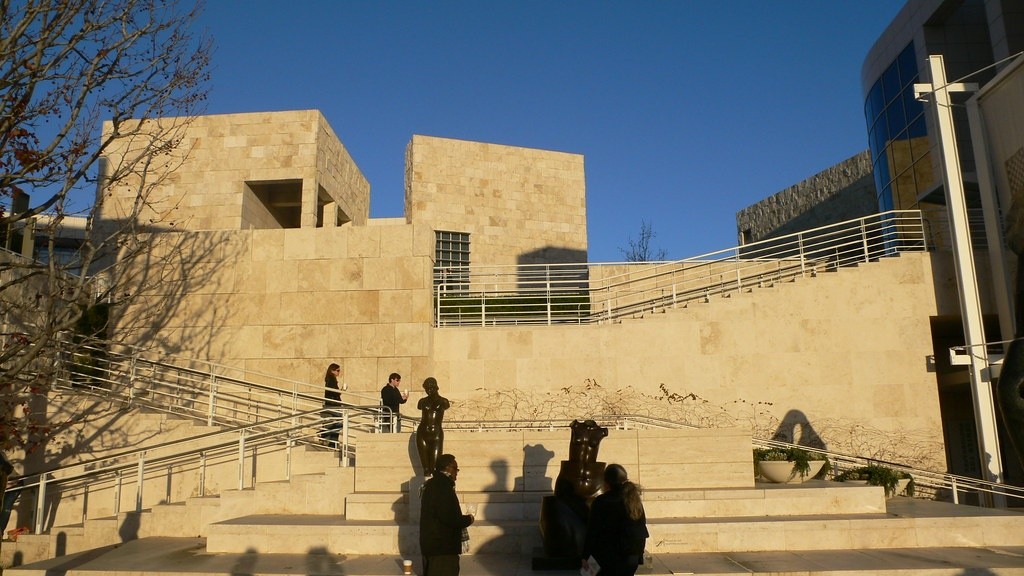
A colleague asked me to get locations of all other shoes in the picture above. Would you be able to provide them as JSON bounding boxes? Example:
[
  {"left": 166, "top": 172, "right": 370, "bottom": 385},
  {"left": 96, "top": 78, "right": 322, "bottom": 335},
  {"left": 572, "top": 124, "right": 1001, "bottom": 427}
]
[
  {"left": 328, "top": 447, "right": 341, "bottom": 452},
  {"left": 317, "top": 431, "right": 326, "bottom": 445}
]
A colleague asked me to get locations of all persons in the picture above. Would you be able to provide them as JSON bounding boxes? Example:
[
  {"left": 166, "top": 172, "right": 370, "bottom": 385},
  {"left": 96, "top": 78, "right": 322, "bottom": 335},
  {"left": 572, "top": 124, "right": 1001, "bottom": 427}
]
[
  {"left": 569, "top": 419, "right": 608, "bottom": 464},
  {"left": 416, "top": 377, "right": 450, "bottom": 477},
  {"left": 381, "top": 373, "right": 409, "bottom": 433},
  {"left": 417, "top": 454, "right": 474, "bottom": 576},
  {"left": 581, "top": 464, "right": 649, "bottom": 576},
  {"left": 316, "top": 363, "right": 347, "bottom": 452}
]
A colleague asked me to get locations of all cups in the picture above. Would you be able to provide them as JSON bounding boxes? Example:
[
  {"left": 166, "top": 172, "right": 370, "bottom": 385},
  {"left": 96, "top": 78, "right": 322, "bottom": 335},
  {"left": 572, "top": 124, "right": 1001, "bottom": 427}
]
[
  {"left": 466, "top": 502, "right": 478, "bottom": 516},
  {"left": 342, "top": 383, "right": 346, "bottom": 391},
  {"left": 403, "top": 560, "right": 412, "bottom": 574},
  {"left": 645, "top": 556, "right": 653, "bottom": 570},
  {"left": 404, "top": 389, "right": 409, "bottom": 397}
]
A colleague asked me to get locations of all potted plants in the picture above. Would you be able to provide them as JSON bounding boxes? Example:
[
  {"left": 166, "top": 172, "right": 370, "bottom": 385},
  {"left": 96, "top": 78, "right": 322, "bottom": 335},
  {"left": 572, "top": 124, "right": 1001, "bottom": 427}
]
[
  {"left": 832, "top": 466, "right": 911, "bottom": 499},
  {"left": 758, "top": 449, "right": 826, "bottom": 485}
]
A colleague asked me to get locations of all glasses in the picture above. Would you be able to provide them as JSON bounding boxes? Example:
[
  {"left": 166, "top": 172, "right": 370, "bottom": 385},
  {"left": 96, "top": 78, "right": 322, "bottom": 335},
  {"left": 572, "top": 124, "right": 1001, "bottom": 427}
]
[
  {"left": 335, "top": 370, "right": 340, "bottom": 372},
  {"left": 451, "top": 466, "right": 459, "bottom": 472}
]
